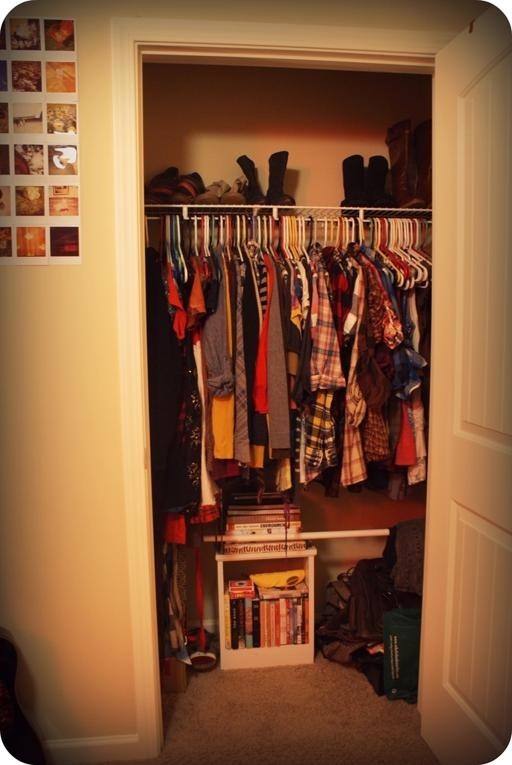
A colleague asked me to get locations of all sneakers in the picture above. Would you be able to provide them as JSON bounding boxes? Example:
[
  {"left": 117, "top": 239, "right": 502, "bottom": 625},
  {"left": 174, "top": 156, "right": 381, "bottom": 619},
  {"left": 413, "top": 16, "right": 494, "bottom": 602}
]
[
  {"left": 193, "top": 177, "right": 231, "bottom": 204},
  {"left": 147, "top": 167, "right": 178, "bottom": 205},
  {"left": 219, "top": 173, "right": 247, "bottom": 205},
  {"left": 172, "top": 168, "right": 206, "bottom": 205}
]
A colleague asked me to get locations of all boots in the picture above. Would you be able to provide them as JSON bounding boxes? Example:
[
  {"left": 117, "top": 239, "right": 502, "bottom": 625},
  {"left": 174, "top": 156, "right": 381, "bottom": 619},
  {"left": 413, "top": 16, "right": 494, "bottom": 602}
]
[
  {"left": 339, "top": 153, "right": 371, "bottom": 216},
  {"left": 384, "top": 118, "right": 428, "bottom": 210},
  {"left": 410, "top": 118, "right": 433, "bottom": 211},
  {"left": 265, "top": 149, "right": 296, "bottom": 213},
  {"left": 235, "top": 153, "right": 265, "bottom": 205},
  {"left": 365, "top": 152, "right": 400, "bottom": 216}
]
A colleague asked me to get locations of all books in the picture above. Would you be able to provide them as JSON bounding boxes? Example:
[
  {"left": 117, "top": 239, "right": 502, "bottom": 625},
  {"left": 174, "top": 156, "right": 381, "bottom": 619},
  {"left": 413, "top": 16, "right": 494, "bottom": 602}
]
[
  {"left": 221, "top": 490, "right": 306, "bottom": 554},
  {"left": 225, "top": 577, "right": 310, "bottom": 650}
]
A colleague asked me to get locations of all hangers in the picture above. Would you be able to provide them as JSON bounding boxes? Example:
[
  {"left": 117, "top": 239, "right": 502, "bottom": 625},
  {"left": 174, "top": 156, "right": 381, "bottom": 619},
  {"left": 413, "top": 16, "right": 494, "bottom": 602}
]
[{"left": 144, "top": 211, "right": 432, "bottom": 292}]
